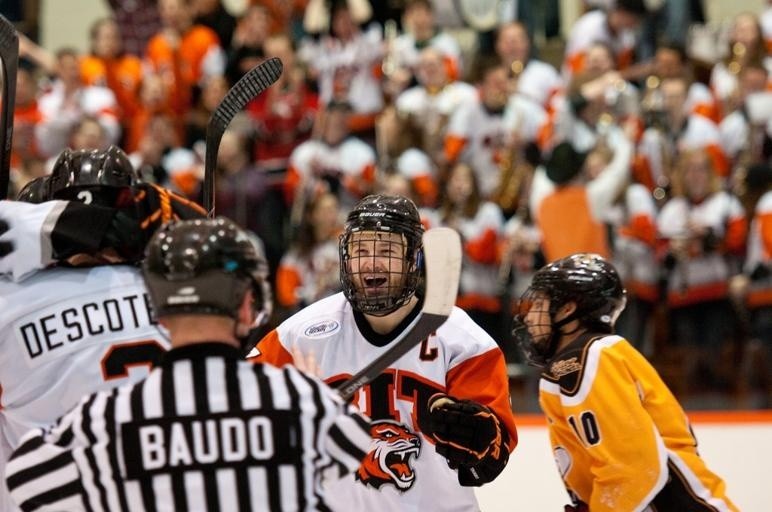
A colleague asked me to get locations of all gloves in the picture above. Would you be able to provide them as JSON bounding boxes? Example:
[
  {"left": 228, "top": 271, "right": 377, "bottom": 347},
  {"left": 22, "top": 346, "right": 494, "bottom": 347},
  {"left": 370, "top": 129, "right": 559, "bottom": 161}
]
[{"left": 431, "top": 399, "right": 512, "bottom": 487}]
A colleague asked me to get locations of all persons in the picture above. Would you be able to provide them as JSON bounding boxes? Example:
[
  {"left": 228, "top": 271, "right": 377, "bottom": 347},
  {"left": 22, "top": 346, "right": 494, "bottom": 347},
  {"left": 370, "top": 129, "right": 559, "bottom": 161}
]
[
  {"left": 241, "top": 195, "right": 518, "bottom": 509},
  {"left": 0, "top": 150, "right": 375, "bottom": 512},
  {"left": 0, "top": 0, "right": 772, "bottom": 415},
  {"left": 512, "top": 253, "right": 742, "bottom": 511}
]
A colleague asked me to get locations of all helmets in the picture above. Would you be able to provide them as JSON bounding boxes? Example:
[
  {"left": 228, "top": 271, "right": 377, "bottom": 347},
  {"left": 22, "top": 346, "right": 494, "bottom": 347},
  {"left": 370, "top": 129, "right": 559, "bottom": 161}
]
[
  {"left": 337, "top": 192, "right": 426, "bottom": 318},
  {"left": 510, "top": 251, "right": 628, "bottom": 370},
  {"left": 15, "top": 142, "right": 143, "bottom": 215},
  {"left": 138, "top": 216, "right": 274, "bottom": 330}
]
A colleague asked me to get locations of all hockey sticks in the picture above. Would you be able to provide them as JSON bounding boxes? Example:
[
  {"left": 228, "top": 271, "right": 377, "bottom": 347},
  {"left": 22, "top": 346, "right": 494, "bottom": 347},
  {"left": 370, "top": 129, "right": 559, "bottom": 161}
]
[
  {"left": 205, "top": 58, "right": 283, "bottom": 220},
  {"left": 1, "top": 17, "right": 20, "bottom": 199}
]
